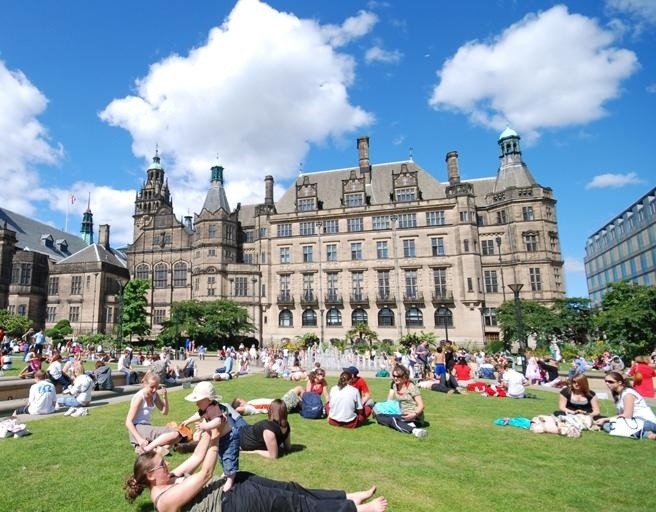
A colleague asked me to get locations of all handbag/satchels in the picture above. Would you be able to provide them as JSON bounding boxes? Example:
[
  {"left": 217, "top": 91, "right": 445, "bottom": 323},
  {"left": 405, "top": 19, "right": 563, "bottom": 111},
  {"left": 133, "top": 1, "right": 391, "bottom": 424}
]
[{"left": 636, "top": 373, "right": 641, "bottom": 382}]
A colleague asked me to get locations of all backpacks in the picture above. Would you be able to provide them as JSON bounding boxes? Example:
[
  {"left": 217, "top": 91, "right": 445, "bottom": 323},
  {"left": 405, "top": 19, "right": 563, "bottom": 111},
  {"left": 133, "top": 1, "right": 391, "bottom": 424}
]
[{"left": 300, "top": 392, "right": 323, "bottom": 418}]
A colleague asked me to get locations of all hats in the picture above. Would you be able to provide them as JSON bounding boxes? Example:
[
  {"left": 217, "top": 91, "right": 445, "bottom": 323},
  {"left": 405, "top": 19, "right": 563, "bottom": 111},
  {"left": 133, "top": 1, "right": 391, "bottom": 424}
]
[
  {"left": 651, "top": 352, "right": 656, "bottom": 360},
  {"left": 343, "top": 366, "right": 359, "bottom": 375},
  {"left": 185, "top": 381, "right": 215, "bottom": 402}
]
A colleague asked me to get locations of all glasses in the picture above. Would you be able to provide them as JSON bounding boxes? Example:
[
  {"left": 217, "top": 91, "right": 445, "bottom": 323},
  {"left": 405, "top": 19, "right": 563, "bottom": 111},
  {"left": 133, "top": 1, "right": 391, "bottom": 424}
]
[
  {"left": 605, "top": 379, "right": 618, "bottom": 383},
  {"left": 392, "top": 375, "right": 400, "bottom": 378}
]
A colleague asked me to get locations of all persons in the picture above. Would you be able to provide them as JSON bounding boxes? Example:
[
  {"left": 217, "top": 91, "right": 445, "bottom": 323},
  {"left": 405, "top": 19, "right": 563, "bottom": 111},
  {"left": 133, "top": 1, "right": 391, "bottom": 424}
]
[
  {"left": 125, "top": 368, "right": 187, "bottom": 456},
  {"left": 555, "top": 372, "right": 656, "bottom": 438},
  {"left": 418, "top": 340, "right": 656, "bottom": 406},
  {"left": 178, "top": 381, "right": 248, "bottom": 493},
  {"left": 176, "top": 399, "right": 291, "bottom": 459},
  {"left": 0, "top": 325, "right": 427, "bottom": 440},
  {"left": 123, "top": 418, "right": 388, "bottom": 512}
]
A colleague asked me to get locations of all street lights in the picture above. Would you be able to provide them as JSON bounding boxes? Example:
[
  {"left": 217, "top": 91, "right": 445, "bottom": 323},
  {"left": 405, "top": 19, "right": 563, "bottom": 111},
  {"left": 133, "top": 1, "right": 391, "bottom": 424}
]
[
  {"left": 507, "top": 283, "right": 526, "bottom": 343},
  {"left": 114, "top": 277, "right": 130, "bottom": 359}
]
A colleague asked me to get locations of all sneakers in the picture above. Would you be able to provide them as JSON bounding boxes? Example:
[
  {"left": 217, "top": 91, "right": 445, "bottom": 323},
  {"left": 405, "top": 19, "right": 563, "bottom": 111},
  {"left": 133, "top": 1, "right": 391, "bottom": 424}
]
[
  {"left": 70, "top": 407, "right": 89, "bottom": 417},
  {"left": 412, "top": 428, "right": 428, "bottom": 438},
  {"left": 65, "top": 407, "right": 77, "bottom": 416}
]
